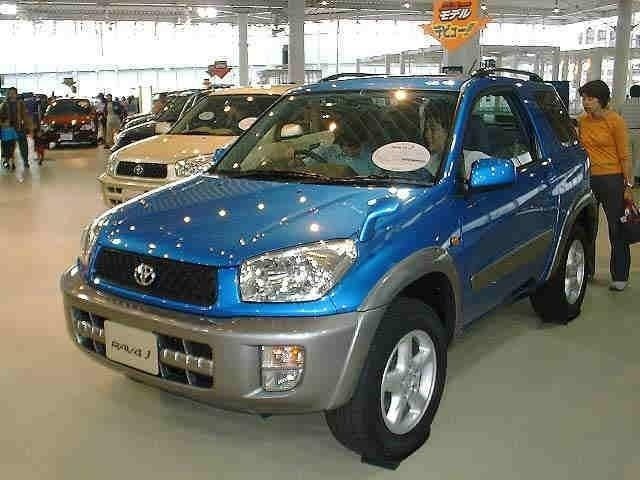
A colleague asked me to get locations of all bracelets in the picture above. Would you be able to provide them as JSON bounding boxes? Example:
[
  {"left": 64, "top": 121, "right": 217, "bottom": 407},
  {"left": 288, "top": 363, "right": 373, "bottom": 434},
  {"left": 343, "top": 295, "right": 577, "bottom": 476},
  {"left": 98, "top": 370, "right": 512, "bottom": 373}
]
[{"left": 624, "top": 178, "right": 632, "bottom": 187}]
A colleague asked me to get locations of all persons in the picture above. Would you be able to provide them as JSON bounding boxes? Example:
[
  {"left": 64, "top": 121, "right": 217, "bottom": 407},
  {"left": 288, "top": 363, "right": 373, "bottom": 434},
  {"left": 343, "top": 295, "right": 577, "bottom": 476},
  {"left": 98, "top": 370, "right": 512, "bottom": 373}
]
[
  {"left": 423, "top": 98, "right": 493, "bottom": 182},
  {"left": 568, "top": 78, "right": 634, "bottom": 291},
  {"left": 94, "top": 93, "right": 138, "bottom": 149},
  {"left": 0, "top": 87, "right": 33, "bottom": 168},
  {"left": 211, "top": 96, "right": 253, "bottom": 130},
  {"left": 282, "top": 118, "right": 387, "bottom": 180},
  {"left": 33, "top": 119, "right": 53, "bottom": 165}
]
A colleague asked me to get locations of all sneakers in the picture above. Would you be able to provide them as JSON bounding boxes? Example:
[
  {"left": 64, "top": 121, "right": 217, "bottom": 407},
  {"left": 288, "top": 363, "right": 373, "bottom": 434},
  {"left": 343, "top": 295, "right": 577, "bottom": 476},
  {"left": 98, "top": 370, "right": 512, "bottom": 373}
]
[{"left": 609, "top": 280, "right": 629, "bottom": 291}]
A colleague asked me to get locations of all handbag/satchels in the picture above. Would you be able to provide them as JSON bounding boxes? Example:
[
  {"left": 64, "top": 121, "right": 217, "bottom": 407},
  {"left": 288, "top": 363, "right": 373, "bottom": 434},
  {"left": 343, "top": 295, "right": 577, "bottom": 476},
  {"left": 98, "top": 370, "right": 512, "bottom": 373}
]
[
  {"left": 619, "top": 205, "right": 640, "bottom": 243},
  {"left": 1, "top": 126, "right": 18, "bottom": 142}
]
